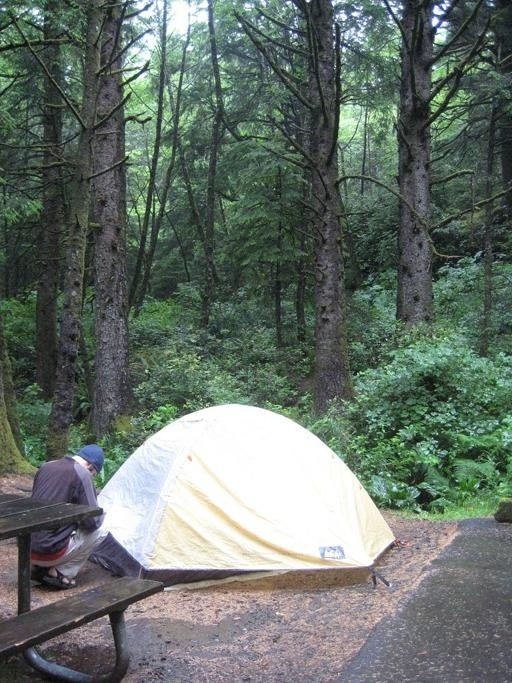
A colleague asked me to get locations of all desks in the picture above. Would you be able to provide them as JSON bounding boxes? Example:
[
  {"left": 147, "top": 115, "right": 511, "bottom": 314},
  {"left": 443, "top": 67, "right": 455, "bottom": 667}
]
[{"left": 0, "top": 493, "right": 130, "bottom": 683}]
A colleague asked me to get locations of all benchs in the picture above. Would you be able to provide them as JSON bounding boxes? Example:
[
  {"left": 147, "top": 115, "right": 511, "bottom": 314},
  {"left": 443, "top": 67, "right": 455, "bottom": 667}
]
[{"left": 0, "top": 575, "right": 165, "bottom": 682}]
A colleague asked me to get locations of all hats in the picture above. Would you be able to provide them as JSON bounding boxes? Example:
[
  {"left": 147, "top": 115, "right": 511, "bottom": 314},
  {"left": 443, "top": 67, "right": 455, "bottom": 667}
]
[{"left": 76, "top": 443, "right": 105, "bottom": 474}]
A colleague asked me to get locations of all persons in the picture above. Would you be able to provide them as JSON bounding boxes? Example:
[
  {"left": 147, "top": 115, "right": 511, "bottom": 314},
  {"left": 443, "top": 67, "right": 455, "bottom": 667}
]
[{"left": 29, "top": 442, "right": 106, "bottom": 587}]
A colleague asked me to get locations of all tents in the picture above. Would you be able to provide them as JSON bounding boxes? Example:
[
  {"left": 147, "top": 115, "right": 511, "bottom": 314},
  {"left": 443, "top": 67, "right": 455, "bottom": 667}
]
[{"left": 85, "top": 401, "right": 401, "bottom": 593}]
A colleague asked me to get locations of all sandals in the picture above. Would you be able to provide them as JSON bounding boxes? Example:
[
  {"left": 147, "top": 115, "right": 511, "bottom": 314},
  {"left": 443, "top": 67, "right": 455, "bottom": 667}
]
[{"left": 29, "top": 566, "right": 77, "bottom": 587}]
